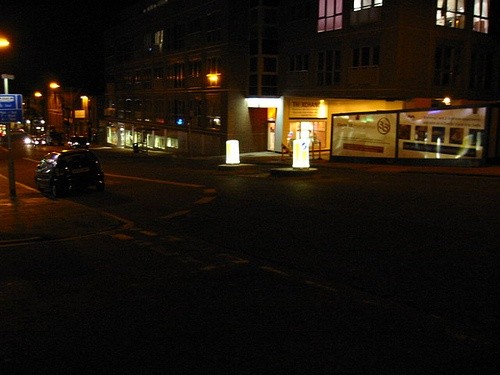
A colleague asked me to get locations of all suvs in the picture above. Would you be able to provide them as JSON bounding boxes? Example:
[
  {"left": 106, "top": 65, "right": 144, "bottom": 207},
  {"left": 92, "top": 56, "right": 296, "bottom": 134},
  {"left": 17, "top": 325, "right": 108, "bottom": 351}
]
[{"left": 35, "top": 148, "right": 104, "bottom": 199}]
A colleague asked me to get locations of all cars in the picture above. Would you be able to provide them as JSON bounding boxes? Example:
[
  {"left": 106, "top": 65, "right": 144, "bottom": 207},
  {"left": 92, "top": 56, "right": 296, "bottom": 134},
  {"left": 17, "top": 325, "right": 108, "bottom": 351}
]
[{"left": 1, "top": 122, "right": 90, "bottom": 152}]
